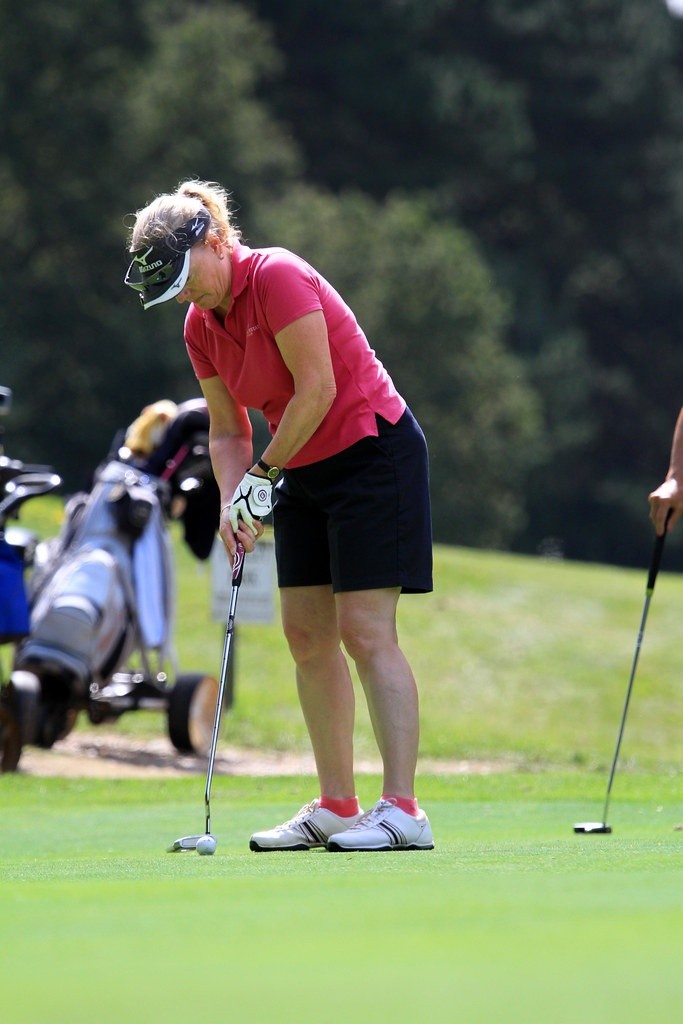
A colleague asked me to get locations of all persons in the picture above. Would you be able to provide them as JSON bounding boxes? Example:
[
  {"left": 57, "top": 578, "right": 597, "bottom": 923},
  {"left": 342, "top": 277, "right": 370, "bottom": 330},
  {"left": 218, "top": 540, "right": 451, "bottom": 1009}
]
[
  {"left": 648, "top": 404, "right": 683, "bottom": 538},
  {"left": 124, "top": 178, "right": 435, "bottom": 853}
]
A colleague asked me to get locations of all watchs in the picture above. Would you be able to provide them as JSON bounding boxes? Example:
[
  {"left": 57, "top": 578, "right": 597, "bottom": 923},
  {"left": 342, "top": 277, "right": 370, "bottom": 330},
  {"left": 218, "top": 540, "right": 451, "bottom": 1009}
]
[{"left": 256, "top": 459, "right": 282, "bottom": 479}]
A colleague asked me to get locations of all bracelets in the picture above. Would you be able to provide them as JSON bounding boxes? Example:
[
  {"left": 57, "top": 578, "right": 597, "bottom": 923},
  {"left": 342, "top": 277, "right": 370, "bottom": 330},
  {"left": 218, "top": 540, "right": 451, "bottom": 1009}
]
[{"left": 221, "top": 504, "right": 231, "bottom": 513}]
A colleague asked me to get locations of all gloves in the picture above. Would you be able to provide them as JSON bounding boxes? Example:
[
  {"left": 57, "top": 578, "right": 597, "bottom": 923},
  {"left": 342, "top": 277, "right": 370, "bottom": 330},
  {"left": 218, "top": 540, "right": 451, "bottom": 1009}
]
[{"left": 229, "top": 472, "right": 273, "bottom": 536}]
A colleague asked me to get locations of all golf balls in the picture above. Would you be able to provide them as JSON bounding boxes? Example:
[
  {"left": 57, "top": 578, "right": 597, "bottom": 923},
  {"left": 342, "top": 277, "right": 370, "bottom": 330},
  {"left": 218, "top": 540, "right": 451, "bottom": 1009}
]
[{"left": 195, "top": 837, "right": 216, "bottom": 856}]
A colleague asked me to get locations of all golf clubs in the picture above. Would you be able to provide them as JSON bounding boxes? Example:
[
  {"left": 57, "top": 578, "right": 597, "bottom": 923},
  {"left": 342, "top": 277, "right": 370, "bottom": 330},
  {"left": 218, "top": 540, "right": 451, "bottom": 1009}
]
[
  {"left": 172, "top": 512, "right": 249, "bottom": 854},
  {"left": 571, "top": 505, "right": 673, "bottom": 834}
]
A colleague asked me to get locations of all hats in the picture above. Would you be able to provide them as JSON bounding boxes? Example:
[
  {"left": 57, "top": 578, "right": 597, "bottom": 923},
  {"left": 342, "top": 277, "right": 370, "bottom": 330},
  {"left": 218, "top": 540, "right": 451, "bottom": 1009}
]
[{"left": 130, "top": 207, "right": 211, "bottom": 310}]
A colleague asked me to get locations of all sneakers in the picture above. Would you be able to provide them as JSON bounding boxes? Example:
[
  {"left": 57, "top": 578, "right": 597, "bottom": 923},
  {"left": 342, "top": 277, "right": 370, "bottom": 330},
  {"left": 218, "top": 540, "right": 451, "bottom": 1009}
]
[
  {"left": 327, "top": 799, "right": 434, "bottom": 851},
  {"left": 250, "top": 799, "right": 365, "bottom": 853}
]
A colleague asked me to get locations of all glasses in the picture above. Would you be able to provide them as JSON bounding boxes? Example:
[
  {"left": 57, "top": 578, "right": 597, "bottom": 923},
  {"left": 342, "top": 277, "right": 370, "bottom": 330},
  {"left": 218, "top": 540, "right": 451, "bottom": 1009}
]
[{"left": 123, "top": 229, "right": 198, "bottom": 293}]
